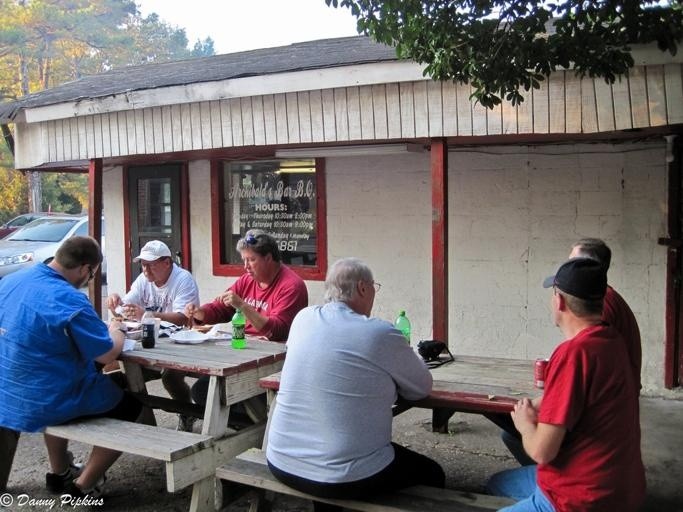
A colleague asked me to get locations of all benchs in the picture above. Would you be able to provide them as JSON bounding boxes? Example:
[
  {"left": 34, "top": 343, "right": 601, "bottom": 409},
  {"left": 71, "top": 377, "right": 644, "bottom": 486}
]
[
  {"left": 215, "top": 446, "right": 518, "bottom": 512},
  {"left": 0, "top": 415, "right": 217, "bottom": 512}
]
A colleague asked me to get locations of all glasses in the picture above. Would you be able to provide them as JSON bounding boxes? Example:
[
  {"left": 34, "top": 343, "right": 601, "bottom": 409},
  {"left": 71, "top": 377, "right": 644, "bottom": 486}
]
[
  {"left": 86, "top": 265, "right": 96, "bottom": 282},
  {"left": 245, "top": 234, "right": 268, "bottom": 249},
  {"left": 357, "top": 278, "right": 381, "bottom": 294}
]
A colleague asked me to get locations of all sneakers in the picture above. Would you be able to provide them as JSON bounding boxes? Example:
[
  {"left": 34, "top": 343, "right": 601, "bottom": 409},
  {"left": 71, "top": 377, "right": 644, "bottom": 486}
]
[
  {"left": 175, "top": 414, "right": 197, "bottom": 432},
  {"left": 46, "top": 461, "right": 85, "bottom": 496},
  {"left": 62, "top": 472, "right": 109, "bottom": 507}
]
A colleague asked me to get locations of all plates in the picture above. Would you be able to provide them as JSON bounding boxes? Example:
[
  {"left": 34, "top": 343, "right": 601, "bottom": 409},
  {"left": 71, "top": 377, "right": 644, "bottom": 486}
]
[
  {"left": 103, "top": 320, "right": 139, "bottom": 328},
  {"left": 167, "top": 333, "right": 229, "bottom": 345}
]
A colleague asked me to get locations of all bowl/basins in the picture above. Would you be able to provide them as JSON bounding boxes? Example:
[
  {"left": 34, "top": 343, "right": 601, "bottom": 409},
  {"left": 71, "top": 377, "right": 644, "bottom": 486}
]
[{"left": 125, "top": 329, "right": 142, "bottom": 341}]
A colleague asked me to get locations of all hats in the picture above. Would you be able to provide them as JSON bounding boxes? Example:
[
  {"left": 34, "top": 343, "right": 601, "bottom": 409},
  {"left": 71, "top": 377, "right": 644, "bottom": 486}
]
[
  {"left": 542, "top": 257, "right": 609, "bottom": 301},
  {"left": 132, "top": 239, "right": 172, "bottom": 264}
]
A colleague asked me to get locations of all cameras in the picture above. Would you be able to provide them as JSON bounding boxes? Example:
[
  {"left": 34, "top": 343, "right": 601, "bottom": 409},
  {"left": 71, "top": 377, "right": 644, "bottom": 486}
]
[{"left": 417, "top": 340, "right": 445, "bottom": 360}]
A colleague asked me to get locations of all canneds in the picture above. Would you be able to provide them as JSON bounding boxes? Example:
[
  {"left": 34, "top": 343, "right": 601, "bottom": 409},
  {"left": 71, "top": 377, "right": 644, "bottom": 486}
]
[{"left": 532, "top": 358, "right": 549, "bottom": 388}]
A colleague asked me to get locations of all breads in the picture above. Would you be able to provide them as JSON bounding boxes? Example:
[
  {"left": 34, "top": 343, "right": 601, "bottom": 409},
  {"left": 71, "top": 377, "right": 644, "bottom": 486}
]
[{"left": 192, "top": 325, "right": 212, "bottom": 333}]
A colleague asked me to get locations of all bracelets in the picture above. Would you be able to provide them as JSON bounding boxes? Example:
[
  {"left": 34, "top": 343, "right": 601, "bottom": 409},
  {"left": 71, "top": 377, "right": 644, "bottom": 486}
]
[{"left": 112, "top": 326, "right": 127, "bottom": 336}]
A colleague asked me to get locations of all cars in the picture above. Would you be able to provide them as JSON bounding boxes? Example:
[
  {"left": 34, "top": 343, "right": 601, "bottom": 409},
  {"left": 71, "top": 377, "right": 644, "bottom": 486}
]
[
  {"left": 0, "top": 213, "right": 107, "bottom": 292},
  {"left": 0, "top": 208, "right": 67, "bottom": 238}
]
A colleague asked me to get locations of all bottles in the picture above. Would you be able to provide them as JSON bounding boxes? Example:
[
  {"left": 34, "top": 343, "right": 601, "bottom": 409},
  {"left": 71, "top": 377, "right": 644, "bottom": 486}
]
[
  {"left": 392, "top": 310, "right": 411, "bottom": 350},
  {"left": 230, "top": 308, "right": 246, "bottom": 351},
  {"left": 140, "top": 306, "right": 155, "bottom": 349}
]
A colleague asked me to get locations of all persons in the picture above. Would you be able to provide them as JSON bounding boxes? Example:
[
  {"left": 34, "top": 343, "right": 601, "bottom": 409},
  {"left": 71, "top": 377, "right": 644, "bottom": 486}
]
[
  {"left": 265, "top": 256, "right": 445, "bottom": 510},
  {"left": 0, "top": 235, "right": 144, "bottom": 506},
  {"left": 500, "top": 237, "right": 643, "bottom": 465},
  {"left": 106, "top": 239, "right": 201, "bottom": 432},
  {"left": 184, "top": 228, "right": 308, "bottom": 431},
  {"left": 485, "top": 256, "right": 647, "bottom": 511}
]
